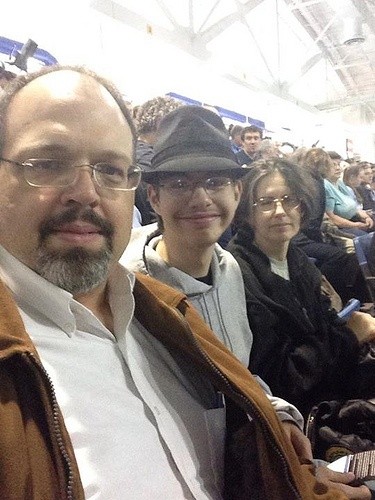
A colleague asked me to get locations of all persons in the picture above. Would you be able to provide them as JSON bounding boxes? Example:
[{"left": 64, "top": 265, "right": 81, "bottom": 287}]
[
  {"left": 226, "top": 120, "right": 375, "bottom": 303},
  {"left": 0, "top": 64, "right": 350, "bottom": 500},
  {"left": 117, "top": 103, "right": 371, "bottom": 500},
  {"left": 225, "top": 158, "right": 375, "bottom": 420},
  {"left": 132, "top": 95, "right": 187, "bottom": 226}
]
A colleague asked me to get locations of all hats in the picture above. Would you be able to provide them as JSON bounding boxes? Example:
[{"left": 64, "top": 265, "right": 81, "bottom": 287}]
[{"left": 140, "top": 106, "right": 253, "bottom": 183}]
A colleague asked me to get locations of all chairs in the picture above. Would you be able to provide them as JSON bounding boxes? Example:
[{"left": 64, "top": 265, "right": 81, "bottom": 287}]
[{"left": 353, "top": 229, "right": 375, "bottom": 304}]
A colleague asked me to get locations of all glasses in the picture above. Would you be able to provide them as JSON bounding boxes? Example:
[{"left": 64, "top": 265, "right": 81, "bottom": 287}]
[
  {"left": 253, "top": 194, "right": 304, "bottom": 211},
  {"left": 0, "top": 157, "right": 144, "bottom": 192},
  {"left": 153, "top": 174, "right": 236, "bottom": 195}
]
